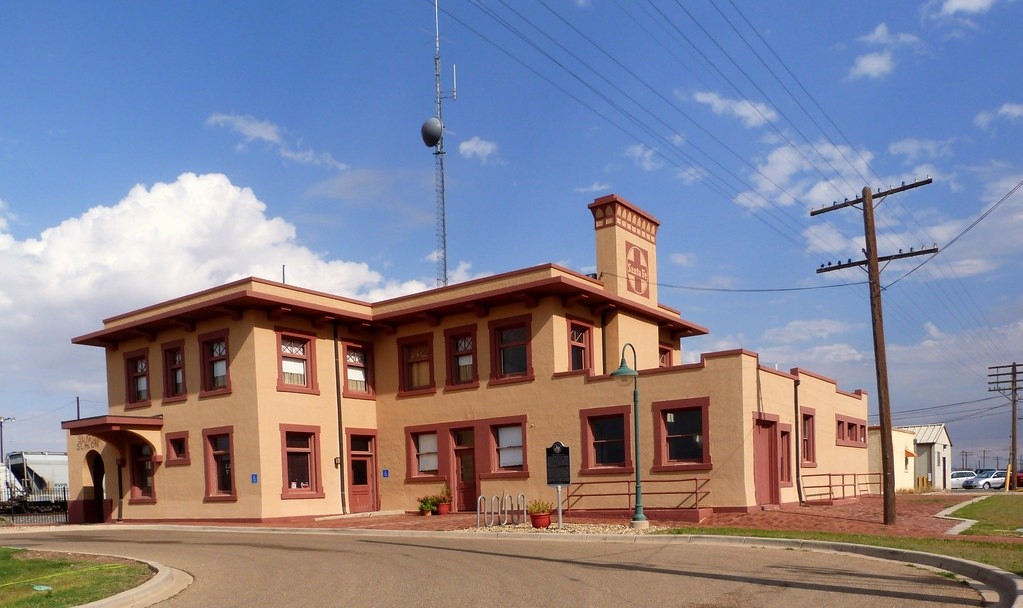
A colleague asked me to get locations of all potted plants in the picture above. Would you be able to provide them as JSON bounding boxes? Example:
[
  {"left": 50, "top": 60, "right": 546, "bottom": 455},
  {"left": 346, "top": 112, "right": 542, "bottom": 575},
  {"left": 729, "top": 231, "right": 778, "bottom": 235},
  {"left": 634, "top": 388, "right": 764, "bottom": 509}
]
[
  {"left": 524, "top": 497, "right": 554, "bottom": 530},
  {"left": 416, "top": 495, "right": 439, "bottom": 517}
]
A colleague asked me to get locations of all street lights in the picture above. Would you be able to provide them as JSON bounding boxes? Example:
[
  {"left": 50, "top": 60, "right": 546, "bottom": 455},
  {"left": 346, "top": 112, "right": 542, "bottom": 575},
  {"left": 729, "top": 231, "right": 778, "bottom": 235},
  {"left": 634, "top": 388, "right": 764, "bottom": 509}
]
[
  {"left": 605, "top": 342, "right": 650, "bottom": 532},
  {"left": 0, "top": 416, "right": 16, "bottom": 462}
]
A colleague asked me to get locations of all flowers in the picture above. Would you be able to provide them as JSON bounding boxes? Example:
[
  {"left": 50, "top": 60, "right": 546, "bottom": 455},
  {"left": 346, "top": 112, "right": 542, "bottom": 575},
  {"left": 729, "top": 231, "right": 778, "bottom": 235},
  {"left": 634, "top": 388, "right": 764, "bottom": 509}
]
[{"left": 433, "top": 494, "right": 454, "bottom": 504}]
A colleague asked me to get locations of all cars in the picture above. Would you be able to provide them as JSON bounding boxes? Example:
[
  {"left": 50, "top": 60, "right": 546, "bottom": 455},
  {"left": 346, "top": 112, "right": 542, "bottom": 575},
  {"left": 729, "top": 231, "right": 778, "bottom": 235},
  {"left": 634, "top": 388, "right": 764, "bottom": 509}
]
[
  {"left": 951, "top": 470, "right": 978, "bottom": 489},
  {"left": 963, "top": 470, "right": 1007, "bottom": 491},
  {"left": 1005, "top": 468, "right": 1023, "bottom": 490},
  {"left": 974, "top": 468, "right": 1006, "bottom": 474}
]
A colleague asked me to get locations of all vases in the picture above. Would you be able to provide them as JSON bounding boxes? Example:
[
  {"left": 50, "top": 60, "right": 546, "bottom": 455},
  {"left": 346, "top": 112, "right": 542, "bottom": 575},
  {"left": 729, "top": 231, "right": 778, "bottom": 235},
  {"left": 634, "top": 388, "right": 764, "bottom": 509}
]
[{"left": 433, "top": 502, "right": 449, "bottom": 515}]
